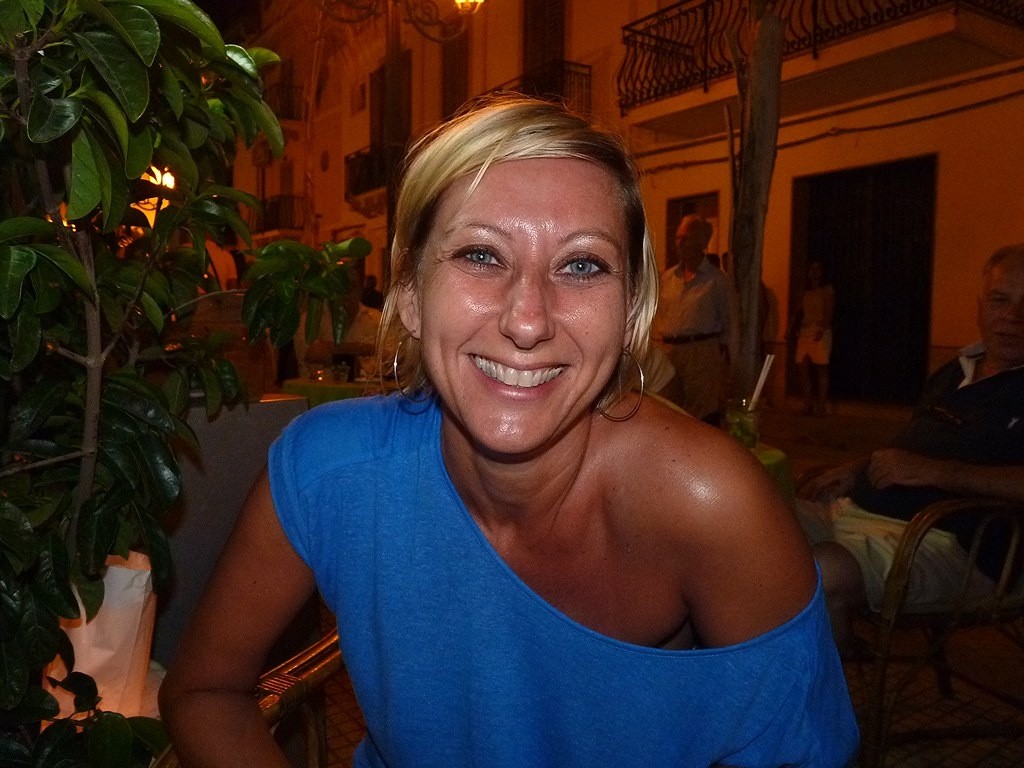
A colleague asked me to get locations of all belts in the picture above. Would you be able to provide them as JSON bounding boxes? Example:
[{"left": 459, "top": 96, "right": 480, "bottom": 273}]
[{"left": 662, "top": 332, "right": 722, "bottom": 345}]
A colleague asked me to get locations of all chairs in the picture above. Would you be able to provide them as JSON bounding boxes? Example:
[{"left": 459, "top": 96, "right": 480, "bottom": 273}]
[{"left": 794, "top": 461, "right": 1024, "bottom": 768}]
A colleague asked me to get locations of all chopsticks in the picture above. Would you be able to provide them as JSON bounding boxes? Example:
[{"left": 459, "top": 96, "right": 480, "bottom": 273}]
[{"left": 749, "top": 355, "right": 774, "bottom": 410}]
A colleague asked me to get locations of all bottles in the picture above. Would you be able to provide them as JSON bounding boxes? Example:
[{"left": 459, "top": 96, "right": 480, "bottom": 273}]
[{"left": 333, "top": 353, "right": 355, "bottom": 383}]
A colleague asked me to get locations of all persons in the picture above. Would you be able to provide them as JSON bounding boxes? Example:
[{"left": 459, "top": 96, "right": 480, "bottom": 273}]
[
  {"left": 156, "top": 98, "right": 862, "bottom": 768},
  {"left": 647, "top": 213, "right": 833, "bottom": 422},
  {"left": 181, "top": 225, "right": 383, "bottom": 382},
  {"left": 791, "top": 243, "right": 1024, "bottom": 704}
]
